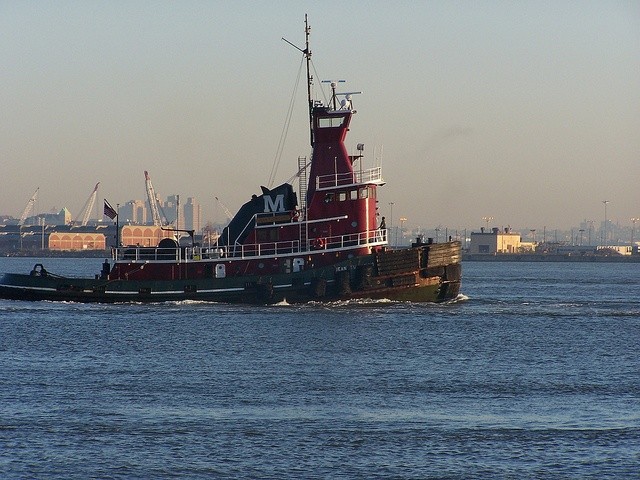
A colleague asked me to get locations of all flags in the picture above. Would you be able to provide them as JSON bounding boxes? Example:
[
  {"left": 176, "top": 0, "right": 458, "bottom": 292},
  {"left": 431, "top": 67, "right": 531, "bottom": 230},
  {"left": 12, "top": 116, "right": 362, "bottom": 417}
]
[{"left": 103, "top": 198, "right": 117, "bottom": 220}]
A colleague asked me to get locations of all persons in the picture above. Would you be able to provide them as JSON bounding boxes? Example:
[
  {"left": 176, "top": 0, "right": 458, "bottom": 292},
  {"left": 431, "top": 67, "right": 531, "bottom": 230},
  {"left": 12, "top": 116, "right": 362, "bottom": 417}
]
[
  {"left": 102, "top": 258, "right": 110, "bottom": 272},
  {"left": 380, "top": 216, "right": 388, "bottom": 241}
]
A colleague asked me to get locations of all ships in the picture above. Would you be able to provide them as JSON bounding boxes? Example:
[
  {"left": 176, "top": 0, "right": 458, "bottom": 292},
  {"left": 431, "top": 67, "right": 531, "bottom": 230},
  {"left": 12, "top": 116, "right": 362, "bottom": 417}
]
[{"left": 1, "top": 10, "right": 464, "bottom": 306}]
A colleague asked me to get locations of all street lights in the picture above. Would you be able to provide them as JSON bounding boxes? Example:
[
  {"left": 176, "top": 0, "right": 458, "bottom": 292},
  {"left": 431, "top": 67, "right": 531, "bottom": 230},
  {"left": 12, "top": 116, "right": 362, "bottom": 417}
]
[
  {"left": 482, "top": 216, "right": 498, "bottom": 230},
  {"left": 601, "top": 199, "right": 612, "bottom": 246},
  {"left": 400, "top": 216, "right": 409, "bottom": 250},
  {"left": 389, "top": 202, "right": 396, "bottom": 246},
  {"left": 29, "top": 198, "right": 39, "bottom": 226},
  {"left": 631, "top": 216, "right": 640, "bottom": 245}
]
